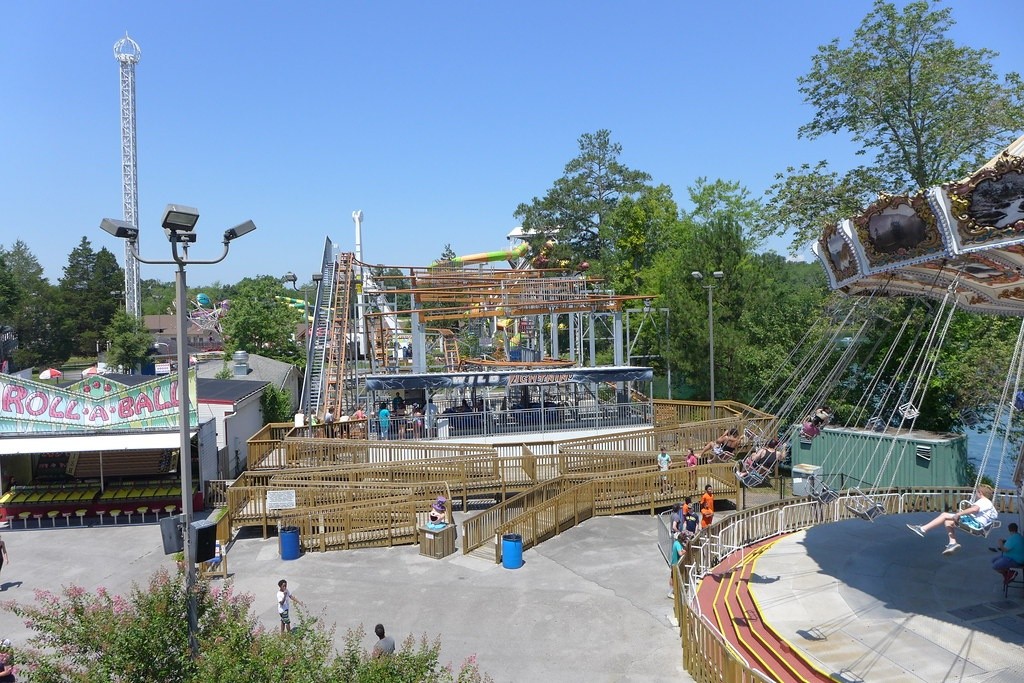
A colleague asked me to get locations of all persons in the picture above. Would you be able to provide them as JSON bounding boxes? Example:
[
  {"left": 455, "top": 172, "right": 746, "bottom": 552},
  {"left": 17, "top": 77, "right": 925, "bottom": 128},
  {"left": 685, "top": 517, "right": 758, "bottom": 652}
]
[
  {"left": 294, "top": 406, "right": 367, "bottom": 439},
  {"left": 701, "top": 428, "right": 787, "bottom": 477},
  {"left": 373, "top": 624, "right": 395, "bottom": 658},
  {"left": 174, "top": 559, "right": 186, "bottom": 592},
  {"left": 1014, "top": 388, "right": 1024, "bottom": 411},
  {"left": 455, "top": 396, "right": 525, "bottom": 412},
  {"left": 378, "top": 392, "right": 438, "bottom": 440},
  {"left": 0, "top": 638, "right": 16, "bottom": 683},
  {"left": 798, "top": 405, "right": 834, "bottom": 440},
  {"left": 276, "top": 580, "right": 302, "bottom": 635},
  {"left": 657, "top": 447, "right": 698, "bottom": 495},
  {"left": 667, "top": 485, "right": 714, "bottom": 600},
  {"left": 6, "top": 476, "right": 18, "bottom": 491},
  {"left": 990, "top": 523, "right": 1024, "bottom": 584},
  {"left": 906, "top": 484, "right": 998, "bottom": 554},
  {"left": 0, "top": 534, "right": 9, "bottom": 591}
]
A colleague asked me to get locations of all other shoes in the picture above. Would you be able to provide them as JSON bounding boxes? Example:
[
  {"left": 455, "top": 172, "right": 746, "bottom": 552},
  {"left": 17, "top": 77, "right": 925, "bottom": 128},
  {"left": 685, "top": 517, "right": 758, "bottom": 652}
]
[
  {"left": 1008, "top": 570, "right": 1018, "bottom": 582},
  {"left": 707, "top": 459, "right": 712, "bottom": 464},
  {"left": 1004, "top": 576, "right": 1009, "bottom": 585},
  {"left": 694, "top": 454, "right": 701, "bottom": 458},
  {"left": 668, "top": 593, "right": 675, "bottom": 599}
]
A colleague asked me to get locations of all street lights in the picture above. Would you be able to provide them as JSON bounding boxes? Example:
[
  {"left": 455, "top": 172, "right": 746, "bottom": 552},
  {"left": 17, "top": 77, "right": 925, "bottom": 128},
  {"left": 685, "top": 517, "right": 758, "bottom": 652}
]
[
  {"left": 286, "top": 272, "right": 324, "bottom": 438},
  {"left": 152, "top": 339, "right": 172, "bottom": 376},
  {"left": 98, "top": 203, "right": 256, "bottom": 668},
  {"left": 109, "top": 290, "right": 127, "bottom": 311},
  {"left": 691, "top": 269, "right": 724, "bottom": 442}
]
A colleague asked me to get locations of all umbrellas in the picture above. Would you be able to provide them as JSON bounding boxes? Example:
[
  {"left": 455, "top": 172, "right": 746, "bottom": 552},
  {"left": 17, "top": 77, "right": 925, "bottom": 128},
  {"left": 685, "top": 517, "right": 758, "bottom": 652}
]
[
  {"left": 81, "top": 367, "right": 104, "bottom": 375},
  {"left": 39, "top": 367, "right": 61, "bottom": 380}
]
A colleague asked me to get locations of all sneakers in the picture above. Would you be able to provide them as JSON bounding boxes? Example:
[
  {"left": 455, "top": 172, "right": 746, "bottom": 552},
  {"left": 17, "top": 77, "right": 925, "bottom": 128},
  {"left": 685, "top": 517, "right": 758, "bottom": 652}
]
[
  {"left": 942, "top": 542, "right": 961, "bottom": 556},
  {"left": 906, "top": 523, "right": 926, "bottom": 538}
]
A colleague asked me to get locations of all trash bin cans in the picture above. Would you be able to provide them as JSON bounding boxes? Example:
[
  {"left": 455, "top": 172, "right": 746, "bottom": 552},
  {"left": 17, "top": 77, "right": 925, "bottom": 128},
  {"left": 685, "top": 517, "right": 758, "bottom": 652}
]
[
  {"left": 280, "top": 526, "right": 300, "bottom": 560},
  {"left": 616, "top": 388, "right": 629, "bottom": 417},
  {"left": 502, "top": 533, "right": 522, "bottom": 569},
  {"left": 437, "top": 419, "right": 449, "bottom": 438}
]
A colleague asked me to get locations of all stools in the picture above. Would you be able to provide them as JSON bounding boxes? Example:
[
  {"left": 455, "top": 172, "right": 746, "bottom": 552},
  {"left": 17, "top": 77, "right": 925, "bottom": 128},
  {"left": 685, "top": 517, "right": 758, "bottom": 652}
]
[
  {"left": 47, "top": 511, "right": 59, "bottom": 527},
  {"left": 62, "top": 512, "right": 71, "bottom": 526},
  {"left": 96, "top": 510, "right": 105, "bottom": 525},
  {"left": 152, "top": 509, "right": 161, "bottom": 521},
  {"left": 137, "top": 507, "right": 148, "bottom": 522},
  {"left": 6, "top": 516, "right": 16, "bottom": 529},
  {"left": 165, "top": 505, "right": 176, "bottom": 517},
  {"left": 19, "top": 512, "right": 32, "bottom": 528},
  {"left": 1003, "top": 564, "right": 1024, "bottom": 598},
  {"left": 75, "top": 510, "right": 88, "bottom": 526},
  {"left": 109, "top": 510, "right": 122, "bottom": 525},
  {"left": 34, "top": 513, "right": 44, "bottom": 527},
  {"left": 124, "top": 511, "right": 134, "bottom": 522}
]
